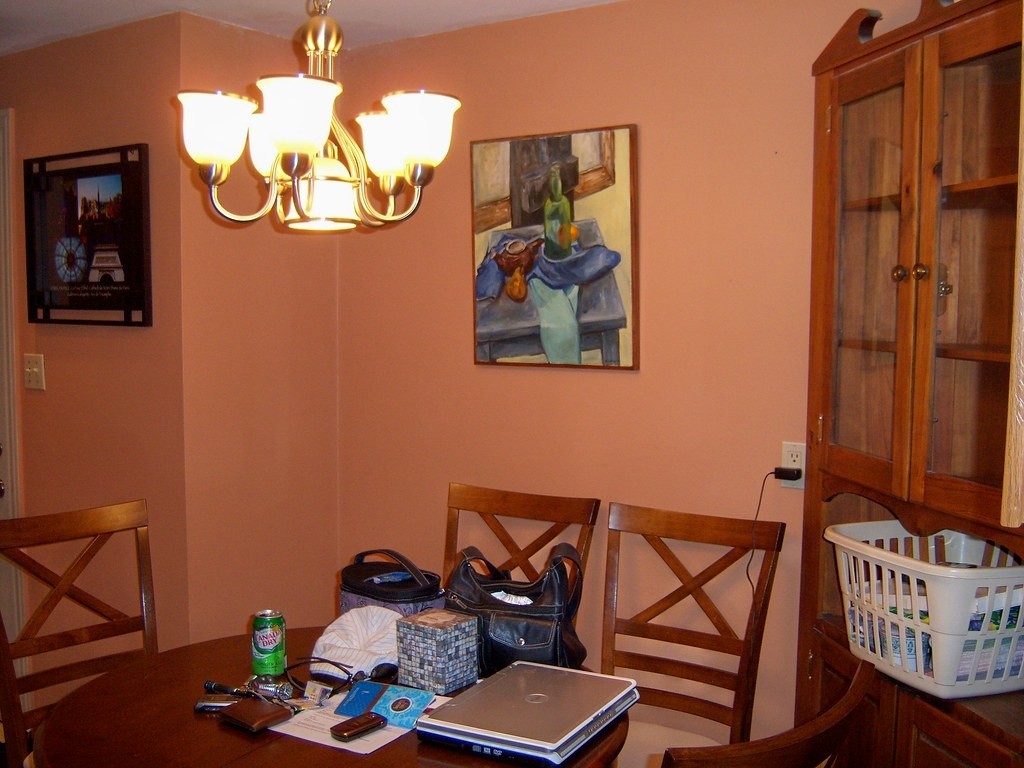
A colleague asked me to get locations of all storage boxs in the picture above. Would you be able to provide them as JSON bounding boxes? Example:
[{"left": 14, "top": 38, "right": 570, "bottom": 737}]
[
  {"left": 821, "top": 518, "right": 1023, "bottom": 700},
  {"left": 396, "top": 607, "right": 481, "bottom": 695}
]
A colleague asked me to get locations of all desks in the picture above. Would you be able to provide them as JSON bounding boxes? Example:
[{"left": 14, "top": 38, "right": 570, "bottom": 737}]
[{"left": 34, "top": 623, "right": 630, "bottom": 768}]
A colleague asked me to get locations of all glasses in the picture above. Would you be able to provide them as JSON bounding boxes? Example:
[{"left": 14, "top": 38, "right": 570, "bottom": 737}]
[
  {"left": 349, "top": 662, "right": 398, "bottom": 692},
  {"left": 284, "top": 657, "right": 353, "bottom": 693}
]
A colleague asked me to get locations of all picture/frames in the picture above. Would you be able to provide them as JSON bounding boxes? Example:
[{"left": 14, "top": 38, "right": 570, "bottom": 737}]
[
  {"left": 23, "top": 143, "right": 152, "bottom": 327},
  {"left": 471, "top": 124, "right": 641, "bottom": 372}
]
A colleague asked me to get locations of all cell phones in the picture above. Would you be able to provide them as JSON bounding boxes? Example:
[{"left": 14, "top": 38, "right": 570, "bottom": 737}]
[{"left": 330, "top": 712, "right": 388, "bottom": 743}]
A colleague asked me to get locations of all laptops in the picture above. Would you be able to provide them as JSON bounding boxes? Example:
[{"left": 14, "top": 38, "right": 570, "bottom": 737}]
[{"left": 416, "top": 661, "right": 640, "bottom": 768}]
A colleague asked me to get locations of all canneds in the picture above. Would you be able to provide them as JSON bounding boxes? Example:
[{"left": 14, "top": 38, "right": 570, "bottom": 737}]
[{"left": 251, "top": 610, "right": 286, "bottom": 676}]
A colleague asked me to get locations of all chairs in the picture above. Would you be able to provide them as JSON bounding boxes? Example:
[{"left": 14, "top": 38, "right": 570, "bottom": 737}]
[
  {"left": 444, "top": 482, "right": 600, "bottom": 628},
  {"left": 661, "top": 658, "right": 876, "bottom": 767},
  {"left": 0, "top": 499, "right": 160, "bottom": 768},
  {"left": 601, "top": 500, "right": 787, "bottom": 748}
]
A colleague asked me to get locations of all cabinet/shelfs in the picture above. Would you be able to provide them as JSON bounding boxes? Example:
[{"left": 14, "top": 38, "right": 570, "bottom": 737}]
[
  {"left": 795, "top": 0, "right": 1022, "bottom": 538},
  {"left": 792, "top": 628, "right": 1024, "bottom": 768}
]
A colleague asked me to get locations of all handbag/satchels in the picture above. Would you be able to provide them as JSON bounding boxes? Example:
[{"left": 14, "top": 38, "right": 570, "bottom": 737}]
[
  {"left": 339, "top": 549, "right": 445, "bottom": 619},
  {"left": 443, "top": 543, "right": 587, "bottom": 669}
]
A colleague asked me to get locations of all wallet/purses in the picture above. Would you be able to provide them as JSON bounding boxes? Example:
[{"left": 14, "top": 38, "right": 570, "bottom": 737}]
[{"left": 218, "top": 696, "right": 292, "bottom": 732}]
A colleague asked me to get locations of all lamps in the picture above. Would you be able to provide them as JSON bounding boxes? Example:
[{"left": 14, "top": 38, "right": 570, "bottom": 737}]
[{"left": 178, "top": 2, "right": 461, "bottom": 235}]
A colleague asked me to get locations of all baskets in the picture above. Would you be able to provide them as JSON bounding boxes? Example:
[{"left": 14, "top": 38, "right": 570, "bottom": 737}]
[{"left": 823, "top": 516, "right": 1023, "bottom": 697}]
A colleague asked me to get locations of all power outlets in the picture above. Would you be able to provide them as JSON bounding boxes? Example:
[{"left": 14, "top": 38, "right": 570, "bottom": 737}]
[{"left": 780, "top": 440, "right": 806, "bottom": 489}]
